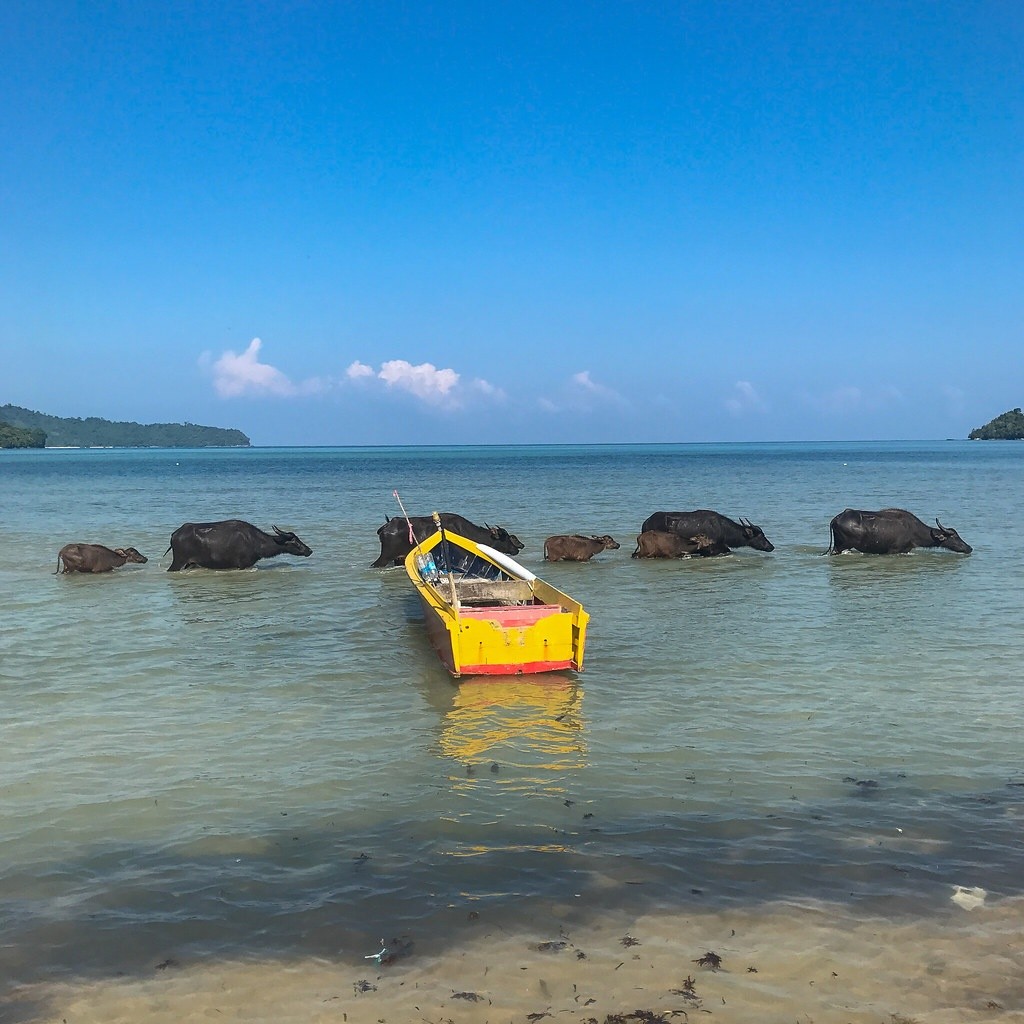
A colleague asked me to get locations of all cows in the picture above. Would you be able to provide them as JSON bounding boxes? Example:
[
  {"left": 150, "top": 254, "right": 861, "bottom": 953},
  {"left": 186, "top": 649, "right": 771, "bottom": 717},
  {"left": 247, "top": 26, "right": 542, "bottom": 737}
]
[
  {"left": 642, "top": 510, "right": 775, "bottom": 556},
  {"left": 162, "top": 519, "right": 315, "bottom": 573},
  {"left": 369, "top": 512, "right": 525, "bottom": 576},
  {"left": 632, "top": 529, "right": 717, "bottom": 560},
  {"left": 820, "top": 506, "right": 973, "bottom": 556},
  {"left": 51, "top": 542, "right": 149, "bottom": 575},
  {"left": 543, "top": 534, "right": 620, "bottom": 563}
]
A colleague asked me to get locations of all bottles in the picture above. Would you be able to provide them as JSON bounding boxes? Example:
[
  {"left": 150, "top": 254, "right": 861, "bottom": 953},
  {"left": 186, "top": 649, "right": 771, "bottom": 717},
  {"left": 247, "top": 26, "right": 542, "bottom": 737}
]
[
  {"left": 416, "top": 555, "right": 431, "bottom": 581},
  {"left": 424, "top": 553, "right": 438, "bottom": 581}
]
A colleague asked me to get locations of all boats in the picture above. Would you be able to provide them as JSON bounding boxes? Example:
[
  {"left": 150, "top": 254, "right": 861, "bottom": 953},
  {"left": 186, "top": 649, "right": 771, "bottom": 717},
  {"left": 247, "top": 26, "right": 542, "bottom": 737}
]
[
  {"left": 402, "top": 508, "right": 589, "bottom": 679},
  {"left": 406, "top": 596, "right": 584, "bottom": 761}
]
